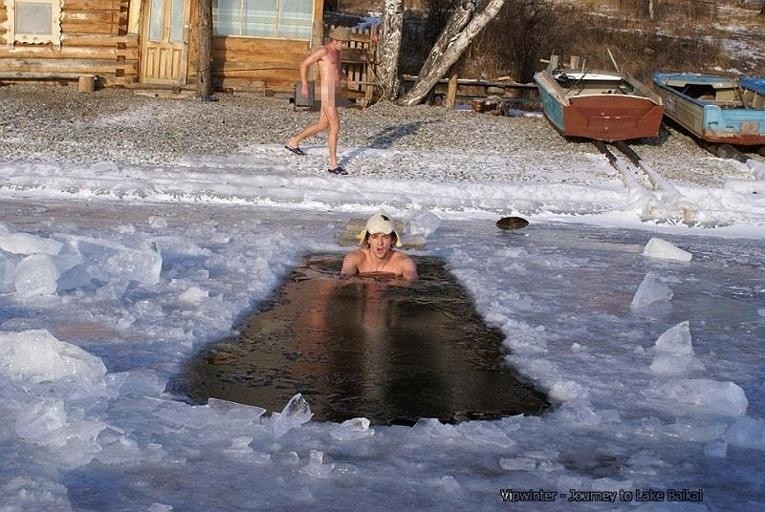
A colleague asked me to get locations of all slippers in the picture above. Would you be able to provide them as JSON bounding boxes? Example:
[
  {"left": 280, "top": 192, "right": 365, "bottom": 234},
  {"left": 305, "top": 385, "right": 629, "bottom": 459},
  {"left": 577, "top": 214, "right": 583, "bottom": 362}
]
[
  {"left": 328, "top": 167, "right": 347, "bottom": 175},
  {"left": 285, "top": 146, "right": 304, "bottom": 155}
]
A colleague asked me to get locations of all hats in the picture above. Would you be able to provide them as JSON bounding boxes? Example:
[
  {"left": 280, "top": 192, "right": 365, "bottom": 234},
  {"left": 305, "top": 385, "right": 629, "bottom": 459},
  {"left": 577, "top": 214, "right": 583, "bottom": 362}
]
[
  {"left": 359, "top": 214, "right": 401, "bottom": 247},
  {"left": 329, "top": 27, "right": 351, "bottom": 41}
]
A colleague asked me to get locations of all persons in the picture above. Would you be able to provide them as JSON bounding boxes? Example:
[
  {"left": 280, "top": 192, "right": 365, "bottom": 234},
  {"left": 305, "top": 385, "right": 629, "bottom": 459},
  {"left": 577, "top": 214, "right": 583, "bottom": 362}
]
[
  {"left": 341, "top": 214, "right": 419, "bottom": 280},
  {"left": 288, "top": 26, "right": 351, "bottom": 175}
]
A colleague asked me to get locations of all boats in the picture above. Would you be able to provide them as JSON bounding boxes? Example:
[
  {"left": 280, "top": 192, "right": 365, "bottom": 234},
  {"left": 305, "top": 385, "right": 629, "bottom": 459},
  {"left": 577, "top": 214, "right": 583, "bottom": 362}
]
[
  {"left": 534, "top": 62, "right": 667, "bottom": 143},
  {"left": 651, "top": 71, "right": 765, "bottom": 147}
]
